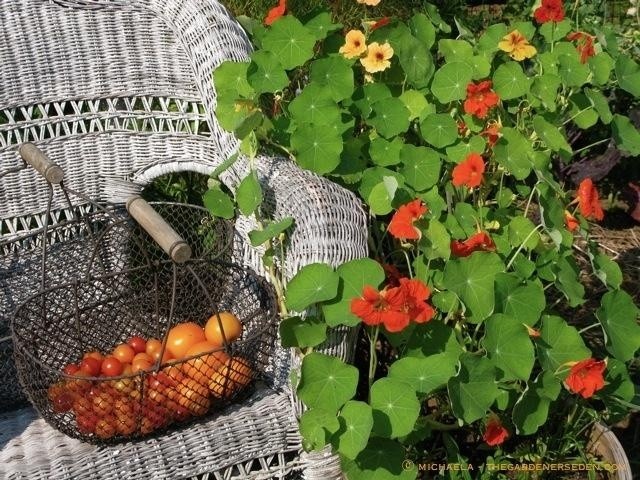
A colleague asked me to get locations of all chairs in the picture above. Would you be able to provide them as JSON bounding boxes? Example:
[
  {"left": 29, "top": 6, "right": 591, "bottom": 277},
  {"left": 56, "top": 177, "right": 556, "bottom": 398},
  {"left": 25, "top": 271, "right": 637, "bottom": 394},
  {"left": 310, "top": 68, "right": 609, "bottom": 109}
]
[{"left": 0, "top": 0, "right": 368, "bottom": 479}]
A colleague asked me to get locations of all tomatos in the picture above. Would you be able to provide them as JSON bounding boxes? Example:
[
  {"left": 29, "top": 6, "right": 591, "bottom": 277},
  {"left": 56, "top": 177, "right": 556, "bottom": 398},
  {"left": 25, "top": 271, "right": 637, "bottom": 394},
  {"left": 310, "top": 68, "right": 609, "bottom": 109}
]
[{"left": 46, "top": 313, "right": 253, "bottom": 439}]
